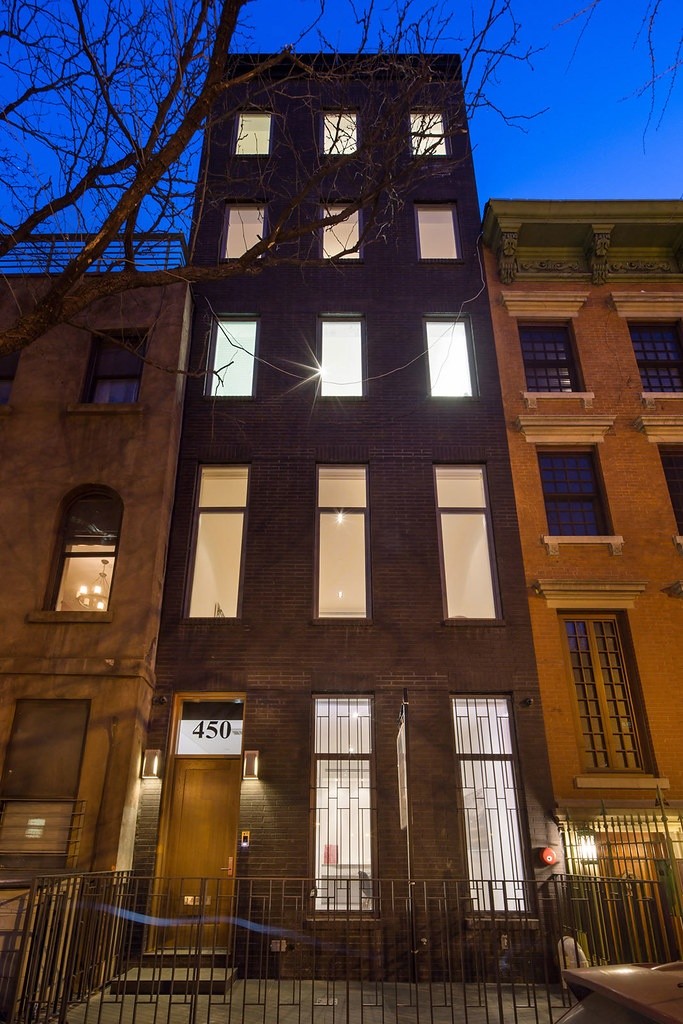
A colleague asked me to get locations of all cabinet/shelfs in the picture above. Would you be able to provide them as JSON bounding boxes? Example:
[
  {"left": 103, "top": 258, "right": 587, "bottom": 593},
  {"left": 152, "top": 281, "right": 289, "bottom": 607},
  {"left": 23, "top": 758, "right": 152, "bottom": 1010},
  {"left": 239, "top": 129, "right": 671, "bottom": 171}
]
[{"left": 464, "top": 786, "right": 497, "bottom": 852}]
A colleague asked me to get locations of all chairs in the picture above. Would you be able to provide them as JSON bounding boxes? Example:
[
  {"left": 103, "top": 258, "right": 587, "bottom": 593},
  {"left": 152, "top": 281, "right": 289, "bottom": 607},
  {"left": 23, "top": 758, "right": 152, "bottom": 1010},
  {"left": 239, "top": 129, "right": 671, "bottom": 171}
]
[{"left": 358, "top": 871, "right": 373, "bottom": 909}]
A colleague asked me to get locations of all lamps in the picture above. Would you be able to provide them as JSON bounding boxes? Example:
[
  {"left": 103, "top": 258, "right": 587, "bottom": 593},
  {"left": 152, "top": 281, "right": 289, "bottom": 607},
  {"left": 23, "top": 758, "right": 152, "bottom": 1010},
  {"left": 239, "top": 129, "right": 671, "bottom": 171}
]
[
  {"left": 77, "top": 559, "right": 110, "bottom": 611},
  {"left": 141, "top": 749, "right": 161, "bottom": 779},
  {"left": 242, "top": 751, "right": 259, "bottom": 779}
]
